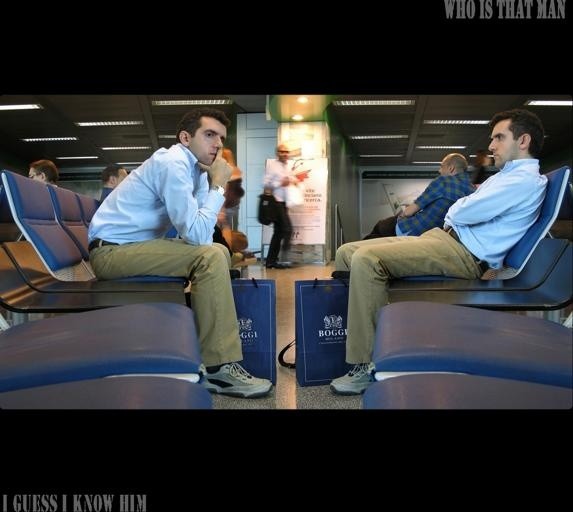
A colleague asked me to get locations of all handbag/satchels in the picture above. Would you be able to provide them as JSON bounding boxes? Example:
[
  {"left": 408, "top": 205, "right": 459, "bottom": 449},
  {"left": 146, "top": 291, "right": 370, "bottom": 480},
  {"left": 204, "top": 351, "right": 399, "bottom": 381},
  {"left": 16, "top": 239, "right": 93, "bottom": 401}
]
[
  {"left": 230, "top": 277, "right": 276, "bottom": 386},
  {"left": 258, "top": 194, "right": 274, "bottom": 225},
  {"left": 294, "top": 278, "right": 354, "bottom": 387}
]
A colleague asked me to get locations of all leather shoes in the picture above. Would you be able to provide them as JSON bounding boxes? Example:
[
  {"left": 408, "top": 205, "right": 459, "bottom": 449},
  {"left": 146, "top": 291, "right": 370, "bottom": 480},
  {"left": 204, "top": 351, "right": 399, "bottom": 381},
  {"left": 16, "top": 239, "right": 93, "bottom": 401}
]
[{"left": 266, "top": 261, "right": 282, "bottom": 269}]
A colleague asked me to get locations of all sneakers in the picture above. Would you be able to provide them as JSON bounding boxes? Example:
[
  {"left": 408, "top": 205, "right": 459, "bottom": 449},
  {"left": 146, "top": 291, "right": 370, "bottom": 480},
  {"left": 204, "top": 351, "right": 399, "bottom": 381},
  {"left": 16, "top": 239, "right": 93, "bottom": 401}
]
[
  {"left": 329, "top": 368, "right": 376, "bottom": 396},
  {"left": 203, "top": 362, "right": 272, "bottom": 398}
]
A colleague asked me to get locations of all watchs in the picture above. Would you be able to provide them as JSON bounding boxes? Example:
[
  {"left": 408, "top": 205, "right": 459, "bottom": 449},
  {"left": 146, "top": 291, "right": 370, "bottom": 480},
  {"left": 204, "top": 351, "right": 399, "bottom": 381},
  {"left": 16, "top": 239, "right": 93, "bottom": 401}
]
[{"left": 210, "top": 183, "right": 224, "bottom": 195}]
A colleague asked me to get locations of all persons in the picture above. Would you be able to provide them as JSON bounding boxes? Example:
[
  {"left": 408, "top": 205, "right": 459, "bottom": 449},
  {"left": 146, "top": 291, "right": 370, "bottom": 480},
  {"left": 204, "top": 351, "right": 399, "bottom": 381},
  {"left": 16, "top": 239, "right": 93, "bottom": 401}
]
[
  {"left": 27, "top": 159, "right": 60, "bottom": 186},
  {"left": 85, "top": 106, "right": 272, "bottom": 399},
  {"left": 328, "top": 108, "right": 548, "bottom": 396},
  {"left": 361, "top": 152, "right": 477, "bottom": 240},
  {"left": 261, "top": 142, "right": 300, "bottom": 269},
  {"left": 97, "top": 165, "right": 128, "bottom": 205}
]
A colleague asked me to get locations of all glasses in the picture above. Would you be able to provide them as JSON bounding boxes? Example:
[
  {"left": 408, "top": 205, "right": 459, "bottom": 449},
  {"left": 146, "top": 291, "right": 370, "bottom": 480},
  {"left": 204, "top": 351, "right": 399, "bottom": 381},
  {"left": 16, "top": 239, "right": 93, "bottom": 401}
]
[{"left": 28, "top": 172, "right": 41, "bottom": 179}]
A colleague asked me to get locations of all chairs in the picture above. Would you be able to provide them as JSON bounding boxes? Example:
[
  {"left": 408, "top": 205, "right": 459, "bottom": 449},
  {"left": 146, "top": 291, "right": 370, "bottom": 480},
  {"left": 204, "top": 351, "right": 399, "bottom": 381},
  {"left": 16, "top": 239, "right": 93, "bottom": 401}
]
[
  {"left": 365, "top": 164, "right": 572, "bottom": 408},
  {"left": 1, "top": 165, "right": 216, "bottom": 410}
]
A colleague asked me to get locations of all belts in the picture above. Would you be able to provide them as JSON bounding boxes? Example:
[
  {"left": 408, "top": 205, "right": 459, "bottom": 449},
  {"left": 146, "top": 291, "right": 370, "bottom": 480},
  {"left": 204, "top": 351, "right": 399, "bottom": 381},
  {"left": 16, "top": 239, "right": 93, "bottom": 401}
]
[
  {"left": 87, "top": 239, "right": 118, "bottom": 253},
  {"left": 445, "top": 226, "right": 488, "bottom": 272}
]
